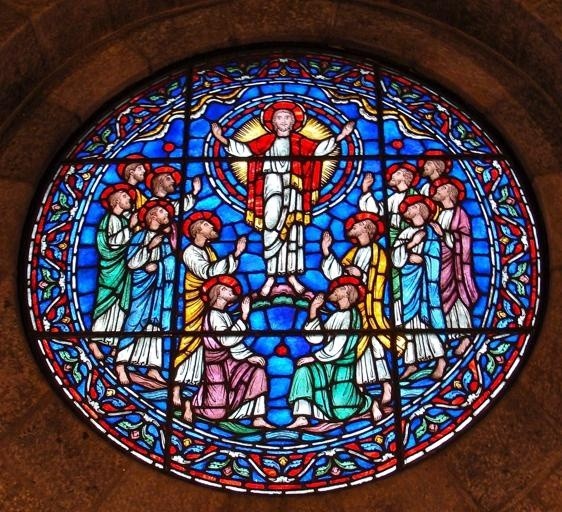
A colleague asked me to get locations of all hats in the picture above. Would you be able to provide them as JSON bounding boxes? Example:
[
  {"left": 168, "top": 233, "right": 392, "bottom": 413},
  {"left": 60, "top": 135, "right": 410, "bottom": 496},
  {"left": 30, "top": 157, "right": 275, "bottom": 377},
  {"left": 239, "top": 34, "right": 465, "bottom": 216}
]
[
  {"left": 144, "top": 164, "right": 183, "bottom": 190},
  {"left": 343, "top": 211, "right": 386, "bottom": 244},
  {"left": 417, "top": 148, "right": 457, "bottom": 176},
  {"left": 384, "top": 161, "right": 420, "bottom": 191},
  {"left": 115, "top": 152, "right": 151, "bottom": 179},
  {"left": 97, "top": 182, "right": 137, "bottom": 211},
  {"left": 428, "top": 175, "right": 467, "bottom": 204},
  {"left": 259, "top": 98, "right": 308, "bottom": 133},
  {"left": 399, "top": 194, "right": 437, "bottom": 224},
  {"left": 199, "top": 274, "right": 242, "bottom": 303},
  {"left": 137, "top": 198, "right": 176, "bottom": 227},
  {"left": 329, "top": 275, "right": 367, "bottom": 304},
  {"left": 181, "top": 210, "right": 222, "bottom": 240}
]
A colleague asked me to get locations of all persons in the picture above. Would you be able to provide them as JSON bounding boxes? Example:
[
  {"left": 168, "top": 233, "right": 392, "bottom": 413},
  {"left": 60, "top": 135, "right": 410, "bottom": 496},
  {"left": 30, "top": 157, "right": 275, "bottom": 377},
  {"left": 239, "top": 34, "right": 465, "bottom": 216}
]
[
  {"left": 86, "top": 181, "right": 138, "bottom": 361},
  {"left": 320, "top": 210, "right": 409, "bottom": 405},
  {"left": 356, "top": 161, "right": 422, "bottom": 329},
  {"left": 211, "top": 99, "right": 356, "bottom": 277},
  {"left": 389, "top": 193, "right": 449, "bottom": 382},
  {"left": 115, "top": 195, "right": 175, "bottom": 387},
  {"left": 115, "top": 151, "right": 151, "bottom": 234},
  {"left": 172, "top": 212, "right": 246, "bottom": 424},
  {"left": 426, "top": 173, "right": 482, "bottom": 356},
  {"left": 284, "top": 274, "right": 383, "bottom": 432},
  {"left": 183, "top": 276, "right": 278, "bottom": 432},
  {"left": 417, "top": 146, "right": 455, "bottom": 197},
  {"left": 144, "top": 164, "right": 201, "bottom": 217}
]
